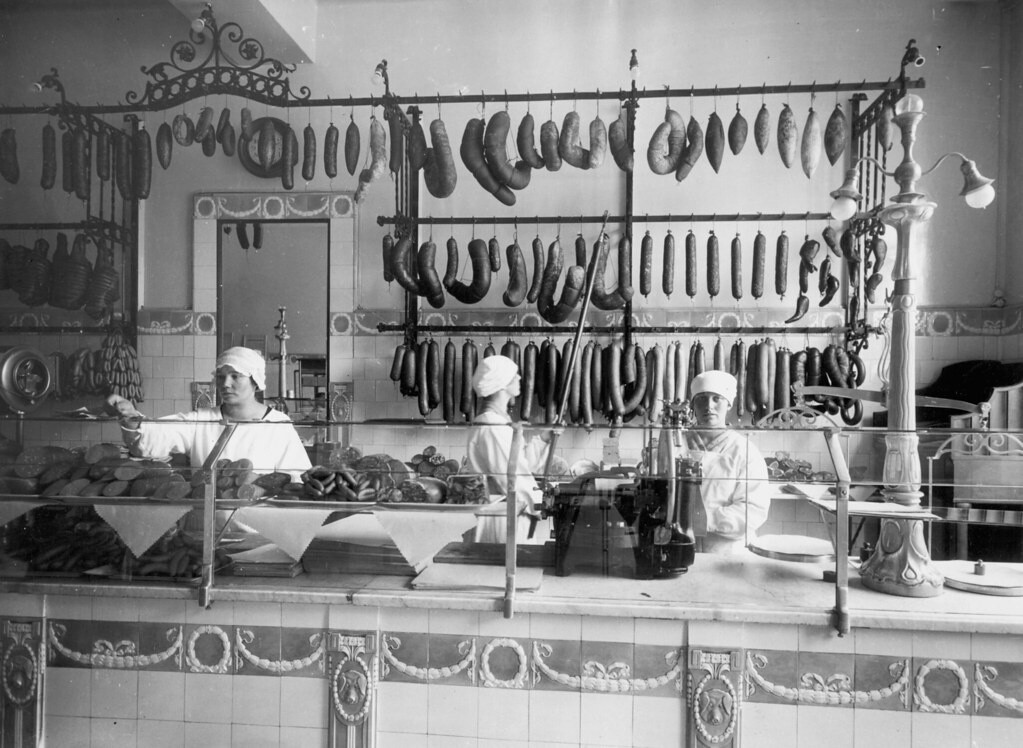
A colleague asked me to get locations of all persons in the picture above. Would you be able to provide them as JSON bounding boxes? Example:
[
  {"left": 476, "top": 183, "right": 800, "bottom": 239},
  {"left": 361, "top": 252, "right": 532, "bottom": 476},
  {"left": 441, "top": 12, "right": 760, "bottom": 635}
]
[
  {"left": 467, "top": 356, "right": 566, "bottom": 544},
  {"left": 105, "top": 347, "right": 312, "bottom": 483},
  {"left": 637, "top": 370, "right": 770, "bottom": 555}
]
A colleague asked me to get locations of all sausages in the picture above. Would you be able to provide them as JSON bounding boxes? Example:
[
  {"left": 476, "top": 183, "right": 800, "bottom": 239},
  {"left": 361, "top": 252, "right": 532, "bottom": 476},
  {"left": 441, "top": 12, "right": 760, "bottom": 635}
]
[
  {"left": 28, "top": 520, "right": 233, "bottom": 581},
  {"left": 297, "top": 461, "right": 385, "bottom": 502},
  {"left": 0, "top": 108, "right": 865, "bottom": 425}
]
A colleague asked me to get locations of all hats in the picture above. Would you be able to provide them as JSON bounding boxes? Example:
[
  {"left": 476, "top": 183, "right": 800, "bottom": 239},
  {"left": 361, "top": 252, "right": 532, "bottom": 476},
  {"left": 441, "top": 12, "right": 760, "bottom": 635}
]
[
  {"left": 473, "top": 355, "right": 518, "bottom": 398},
  {"left": 211, "top": 346, "right": 266, "bottom": 392},
  {"left": 691, "top": 370, "right": 738, "bottom": 410}
]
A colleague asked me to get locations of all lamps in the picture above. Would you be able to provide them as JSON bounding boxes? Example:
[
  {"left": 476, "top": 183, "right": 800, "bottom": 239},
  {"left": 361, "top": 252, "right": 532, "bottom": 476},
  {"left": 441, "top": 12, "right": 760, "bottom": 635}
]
[{"left": 830, "top": 95, "right": 995, "bottom": 597}]
